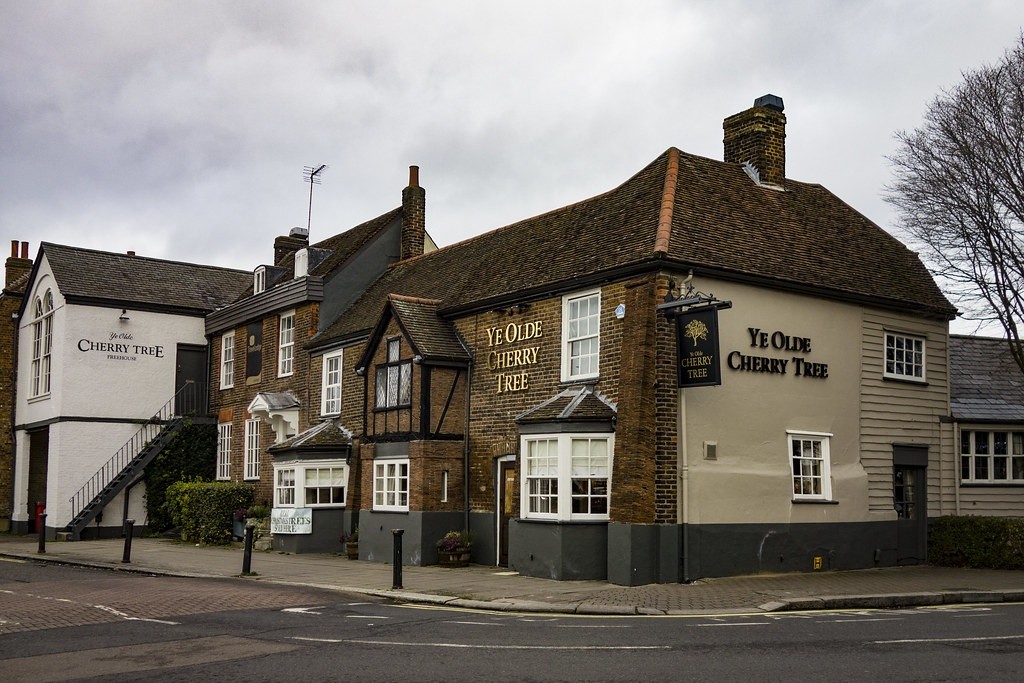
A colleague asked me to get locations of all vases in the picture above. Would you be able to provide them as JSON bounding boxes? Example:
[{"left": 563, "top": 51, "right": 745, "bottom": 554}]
[
  {"left": 344, "top": 542, "right": 359, "bottom": 560},
  {"left": 438, "top": 548, "right": 471, "bottom": 567}
]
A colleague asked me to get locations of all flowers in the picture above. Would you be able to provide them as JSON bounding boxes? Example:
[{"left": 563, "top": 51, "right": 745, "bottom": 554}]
[{"left": 436, "top": 531, "right": 472, "bottom": 548}]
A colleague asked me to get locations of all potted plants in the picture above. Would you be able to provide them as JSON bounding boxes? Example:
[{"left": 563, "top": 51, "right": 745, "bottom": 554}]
[
  {"left": 337, "top": 527, "right": 359, "bottom": 542},
  {"left": 233, "top": 503, "right": 271, "bottom": 535}
]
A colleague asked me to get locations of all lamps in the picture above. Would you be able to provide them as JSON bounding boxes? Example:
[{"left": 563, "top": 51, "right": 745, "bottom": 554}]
[
  {"left": 356, "top": 367, "right": 365, "bottom": 375},
  {"left": 119, "top": 309, "right": 130, "bottom": 320},
  {"left": 413, "top": 355, "right": 422, "bottom": 364}
]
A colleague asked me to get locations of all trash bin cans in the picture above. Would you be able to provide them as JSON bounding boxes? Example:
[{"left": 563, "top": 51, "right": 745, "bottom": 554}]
[{"left": 37, "top": 501, "right": 44, "bottom": 533}]
